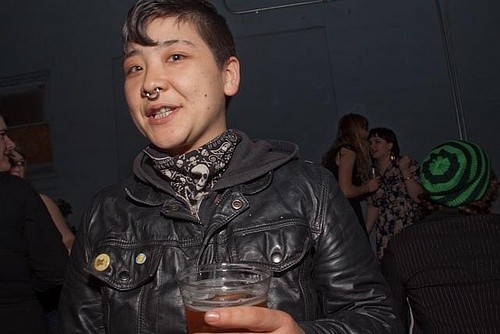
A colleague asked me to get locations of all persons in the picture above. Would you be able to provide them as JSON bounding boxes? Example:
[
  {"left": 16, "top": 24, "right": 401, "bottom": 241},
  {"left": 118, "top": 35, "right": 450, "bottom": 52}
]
[
  {"left": 0, "top": 115, "right": 72, "bottom": 334},
  {"left": 323, "top": 113, "right": 382, "bottom": 235},
  {"left": 386, "top": 139, "right": 500, "bottom": 334},
  {"left": 366, "top": 128, "right": 424, "bottom": 265},
  {"left": 8, "top": 142, "right": 76, "bottom": 256},
  {"left": 61, "top": 0, "right": 401, "bottom": 334}
]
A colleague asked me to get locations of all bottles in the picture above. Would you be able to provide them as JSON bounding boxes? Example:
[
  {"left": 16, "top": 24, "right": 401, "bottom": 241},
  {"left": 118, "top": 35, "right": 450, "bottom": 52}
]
[{"left": 371, "top": 165, "right": 376, "bottom": 179}]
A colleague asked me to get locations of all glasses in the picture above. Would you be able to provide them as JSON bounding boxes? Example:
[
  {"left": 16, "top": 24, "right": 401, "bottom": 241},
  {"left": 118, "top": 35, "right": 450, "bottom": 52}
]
[{"left": 11, "top": 160, "right": 27, "bottom": 167}]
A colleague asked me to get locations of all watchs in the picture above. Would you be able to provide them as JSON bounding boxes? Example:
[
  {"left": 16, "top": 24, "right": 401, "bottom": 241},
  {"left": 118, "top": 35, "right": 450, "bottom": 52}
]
[{"left": 402, "top": 176, "right": 414, "bottom": 180}]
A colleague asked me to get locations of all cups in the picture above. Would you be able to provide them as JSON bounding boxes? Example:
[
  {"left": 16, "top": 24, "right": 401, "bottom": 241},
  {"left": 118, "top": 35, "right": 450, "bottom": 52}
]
[{"left": 174, "top": 262, "right": 274, "bottom": 334}]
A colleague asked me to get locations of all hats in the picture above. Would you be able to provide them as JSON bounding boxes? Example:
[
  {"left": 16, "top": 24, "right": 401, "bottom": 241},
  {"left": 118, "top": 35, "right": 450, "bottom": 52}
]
[{"left": 419, "top": 139, "right": 489, "bottom": 207}]
